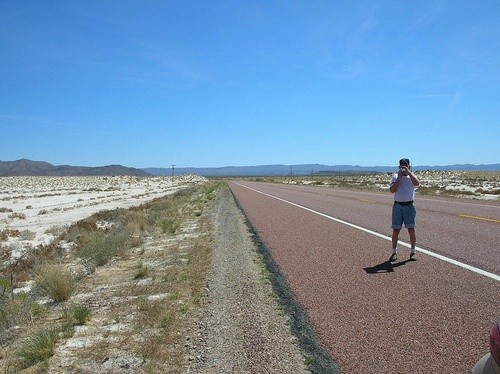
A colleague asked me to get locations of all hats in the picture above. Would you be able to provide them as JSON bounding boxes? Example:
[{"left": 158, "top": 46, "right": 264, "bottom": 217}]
[{"left": 400, "top": 159, "right": 409, "bottom": 165}]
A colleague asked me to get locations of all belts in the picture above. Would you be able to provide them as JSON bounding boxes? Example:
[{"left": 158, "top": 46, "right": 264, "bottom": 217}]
[{"left": 395, "top": 201, "right": 413, "bottom": 205}]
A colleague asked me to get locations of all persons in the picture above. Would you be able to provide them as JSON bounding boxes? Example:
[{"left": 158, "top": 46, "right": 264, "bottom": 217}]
[
  {"left": 472, "top": 317, "right": 500, "bottom": 374},
  {"left": 390, "top": 159, "right": 420, "bottom": 261}
]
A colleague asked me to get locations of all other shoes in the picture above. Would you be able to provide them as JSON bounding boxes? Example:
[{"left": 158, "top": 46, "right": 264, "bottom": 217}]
[
  {"left": 390, "top": 254, "right": 397, "bottom": 260},
  {"left": 410, "top": 253, "right": 417, "bottom": 260}
]
